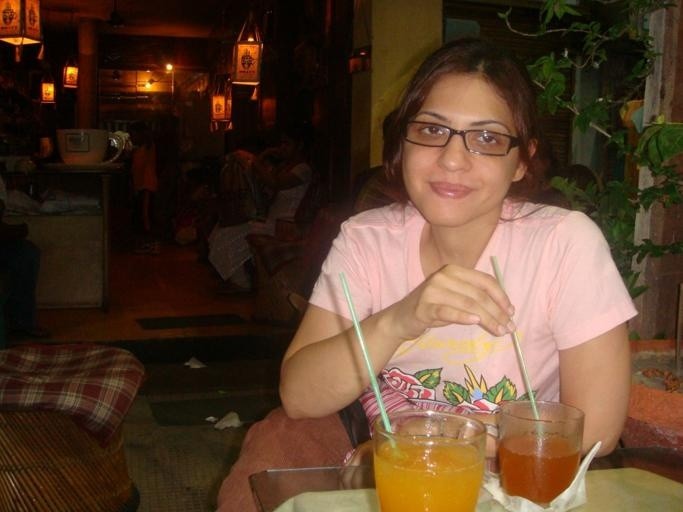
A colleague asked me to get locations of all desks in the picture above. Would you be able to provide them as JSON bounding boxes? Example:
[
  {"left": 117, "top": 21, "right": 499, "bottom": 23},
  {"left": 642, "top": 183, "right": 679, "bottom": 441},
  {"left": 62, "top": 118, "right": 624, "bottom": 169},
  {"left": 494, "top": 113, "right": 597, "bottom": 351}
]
[{"left": 247, "top": 449, "right": 683, "bottom": 512}]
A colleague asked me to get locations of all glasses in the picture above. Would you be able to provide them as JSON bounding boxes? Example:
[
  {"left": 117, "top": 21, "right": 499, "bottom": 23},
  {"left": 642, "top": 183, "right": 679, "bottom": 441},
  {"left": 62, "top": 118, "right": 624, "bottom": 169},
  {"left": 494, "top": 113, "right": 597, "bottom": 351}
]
[{"left": 401, "top": 119, "right": 521, "bottom": 157}]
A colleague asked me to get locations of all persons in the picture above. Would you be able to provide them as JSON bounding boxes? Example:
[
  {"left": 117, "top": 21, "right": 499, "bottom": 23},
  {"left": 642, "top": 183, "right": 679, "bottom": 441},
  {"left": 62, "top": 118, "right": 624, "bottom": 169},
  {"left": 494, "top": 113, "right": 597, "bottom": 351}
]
[
  {"left": 275, "top": 35, "right": 638, "bottom": 492},
  {"left": 117, "top": 122, "right": 312, "bottom": 238}
]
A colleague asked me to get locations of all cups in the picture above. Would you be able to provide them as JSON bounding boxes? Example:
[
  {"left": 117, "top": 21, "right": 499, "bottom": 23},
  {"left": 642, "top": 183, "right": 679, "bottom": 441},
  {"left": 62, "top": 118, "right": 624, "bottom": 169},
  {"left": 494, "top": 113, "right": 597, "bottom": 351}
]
[
  {"left": 55, "top": 127, "right": 123, "bottom": 166},
  {"left": 495, "top": 399, "right": 585, "bottom": 509},
  {"left": 372, "top": 409, "right": 488, "bottom": 512}
]
